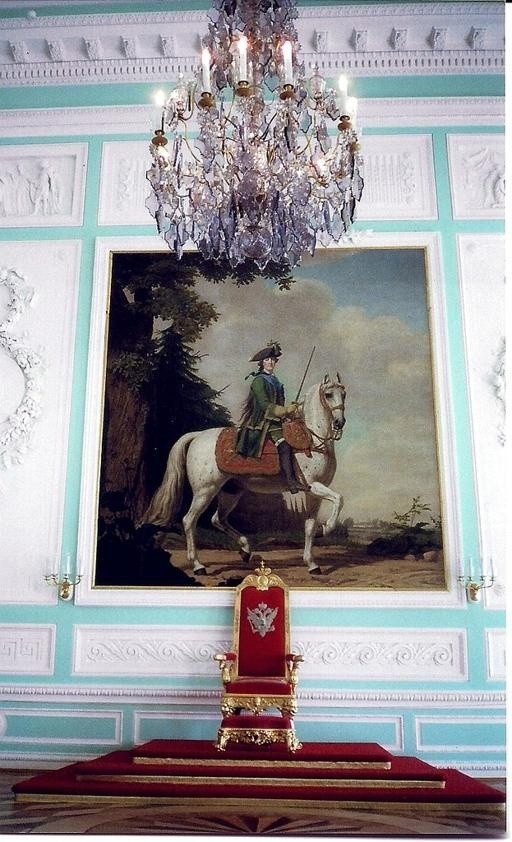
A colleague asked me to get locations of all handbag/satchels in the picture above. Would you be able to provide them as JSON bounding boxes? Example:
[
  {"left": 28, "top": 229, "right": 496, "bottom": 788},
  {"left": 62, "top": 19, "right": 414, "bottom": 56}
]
[{"left": 282, "top": 417, "right": 313, "bottom": 450}]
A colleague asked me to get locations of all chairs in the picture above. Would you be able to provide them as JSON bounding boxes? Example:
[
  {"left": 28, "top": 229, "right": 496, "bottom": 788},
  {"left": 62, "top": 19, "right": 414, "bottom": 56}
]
[{"left": 214, "top": 559, "right": 306, "bottom": 754}]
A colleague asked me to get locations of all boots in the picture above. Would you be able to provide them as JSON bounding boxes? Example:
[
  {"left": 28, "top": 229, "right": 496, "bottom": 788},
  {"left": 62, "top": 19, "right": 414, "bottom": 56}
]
[{"left": 276, "top": 441, "right": 312, "bottom": 494}]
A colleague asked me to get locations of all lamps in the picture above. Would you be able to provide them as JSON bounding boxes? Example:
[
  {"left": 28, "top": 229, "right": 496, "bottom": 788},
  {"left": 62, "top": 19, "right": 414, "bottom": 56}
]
[
  {"left": 456, "top": 555, "right": 495, "bottom": 605},
  {"left": 145, "top": 0, "right": 365, "bottom": 271},
  {"left": 43, "top": 551, "right": 83, "bottom": 600}
]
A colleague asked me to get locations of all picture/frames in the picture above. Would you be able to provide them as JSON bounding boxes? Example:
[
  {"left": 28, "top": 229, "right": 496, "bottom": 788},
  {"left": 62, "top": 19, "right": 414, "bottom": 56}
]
[{"left": 75, "top": 234, "right": 466, "bottom": 611}]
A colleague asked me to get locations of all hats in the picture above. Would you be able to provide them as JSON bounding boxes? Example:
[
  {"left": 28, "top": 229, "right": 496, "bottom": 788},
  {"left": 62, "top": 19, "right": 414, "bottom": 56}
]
[{"left": 248, "top": 339, "right": 282, "bottom": 362}]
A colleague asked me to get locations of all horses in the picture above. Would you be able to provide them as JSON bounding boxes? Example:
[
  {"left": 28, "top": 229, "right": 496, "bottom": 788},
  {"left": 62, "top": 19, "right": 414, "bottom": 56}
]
[{"left": 137, "top": 372, "right": 347, "bottom": 576}]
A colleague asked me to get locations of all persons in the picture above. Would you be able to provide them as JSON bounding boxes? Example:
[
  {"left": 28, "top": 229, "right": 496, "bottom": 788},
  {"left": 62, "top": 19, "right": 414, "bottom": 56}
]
[{"left": 233, "top": 345, "right": 312, "bottom": 494}]
